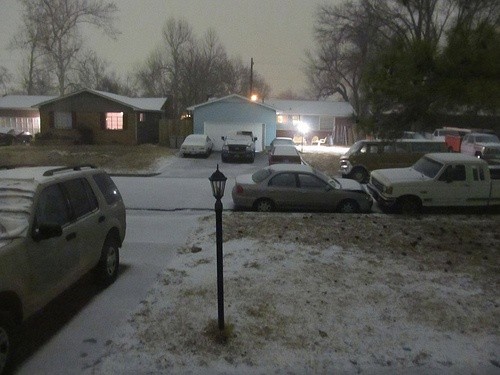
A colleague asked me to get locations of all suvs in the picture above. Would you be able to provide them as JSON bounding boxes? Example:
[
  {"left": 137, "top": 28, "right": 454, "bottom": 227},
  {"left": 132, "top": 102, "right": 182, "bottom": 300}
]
[
  {"left": 221, "top": 133, "right": 257, "bottom": 163},
  {"left": 0, "top": 163, "right": 127, "bottom": 375}
]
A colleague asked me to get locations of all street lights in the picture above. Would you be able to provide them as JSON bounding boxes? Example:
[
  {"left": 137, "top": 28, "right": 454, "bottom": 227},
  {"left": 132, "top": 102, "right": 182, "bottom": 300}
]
[{"left": 208, "top": 163, "right": 228, "bottom": 332}]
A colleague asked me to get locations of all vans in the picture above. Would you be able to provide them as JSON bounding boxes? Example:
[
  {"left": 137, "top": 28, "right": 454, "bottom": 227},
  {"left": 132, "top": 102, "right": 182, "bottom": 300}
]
[{"left": 337, "top": 127, "right": 500, "bottom": 214}]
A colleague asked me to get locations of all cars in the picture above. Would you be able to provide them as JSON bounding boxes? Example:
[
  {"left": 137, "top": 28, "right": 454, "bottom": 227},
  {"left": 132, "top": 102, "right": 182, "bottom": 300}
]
[
  {"left": 267, "top": 137, "right": 302, "bottom": 163},
  {"left": 232, "top": 164, "right": 373, "bottom": 214},
  {"left": 180, "top": 134, "right": 213, "bottom": 158}
]
